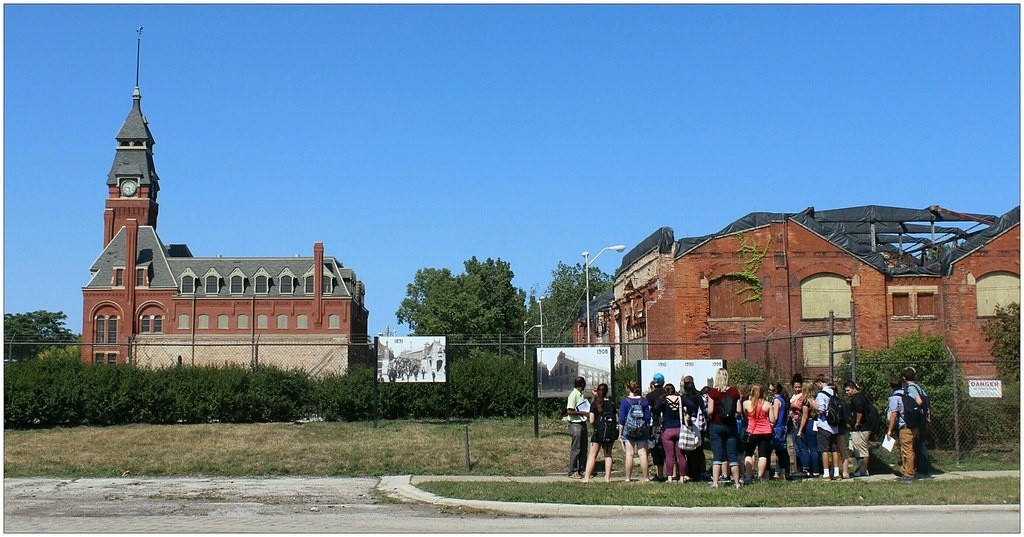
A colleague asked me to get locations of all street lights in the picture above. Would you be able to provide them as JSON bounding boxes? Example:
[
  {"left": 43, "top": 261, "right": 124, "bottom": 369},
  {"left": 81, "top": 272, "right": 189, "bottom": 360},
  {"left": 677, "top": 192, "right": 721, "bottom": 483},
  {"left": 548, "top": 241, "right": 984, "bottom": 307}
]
[
  {"left": 523, "top": 324, "right": 544, "bottom": 364},
  {"left": 583, "top": 246, "right": 626, "bottom": 347}
]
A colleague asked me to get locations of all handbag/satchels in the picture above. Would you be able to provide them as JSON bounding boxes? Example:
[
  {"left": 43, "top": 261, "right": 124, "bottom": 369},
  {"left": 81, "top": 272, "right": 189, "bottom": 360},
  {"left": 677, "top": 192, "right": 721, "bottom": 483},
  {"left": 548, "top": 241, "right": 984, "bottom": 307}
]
[
  {"left": 720, "top": 394, "right": 735, "bottom": 420},
  {"left": 691, "top": 405, "right": 708, "bottom": 432},
  {"left": 678, "top": 396, "right": 702, "bottom": 451},
  {"left": 787, "top": 418, "right": 793, "bottom": 433}
]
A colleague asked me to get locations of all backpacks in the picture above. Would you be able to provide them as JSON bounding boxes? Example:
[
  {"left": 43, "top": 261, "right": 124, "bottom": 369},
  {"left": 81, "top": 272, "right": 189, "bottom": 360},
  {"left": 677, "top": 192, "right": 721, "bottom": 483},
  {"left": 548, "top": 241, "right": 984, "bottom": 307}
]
[
  {"left": 850, "top": 395, "right": 882, "bottom": 434},
  {"left": 623, "top": 397, "right": 645, "bottom": 436},
  {"left": 594, "top": 401, "right": 617, "bottom": 443},
  {"left": 893, "top": 394, "right": 923, "bottom": 428},
  {"left": 909, "top": 385, "right": 930, "bottom": 411},
  {"left": 816, "top": 390, "right": 845, "bottom": 426}
]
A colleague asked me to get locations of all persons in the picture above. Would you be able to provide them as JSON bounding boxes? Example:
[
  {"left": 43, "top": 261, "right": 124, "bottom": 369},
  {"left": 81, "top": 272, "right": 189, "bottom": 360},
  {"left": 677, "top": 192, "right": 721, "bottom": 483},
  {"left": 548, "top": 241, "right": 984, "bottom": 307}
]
[
  {"left": 654, "top": 384, "right": 692, "bottom": 484},
  {"left": 618, "top": 380, "right": 652, "bottom": 483},
  {"left": 700, "top": 386, "right": 731, "bottom": 484},
  {"left": 797, "top": 381, "right": 821, "bottom": 478},
  {"left": 705, "top": 368, "right": 743, "bottom": 488},
  {"left": 790, "top": 373, "right": 812, "bottom": 478},
  {"left": 580, "top": 383, "right": 618, "bottom": 484},
  {"left": 738, "top": 384, "right": 775, "bottom": 484},
  {"left": 647, "top": 373, "right": 671, "bottom": 483},
  {"left": 567, "top": 377, "right": 597, "bottom": 479},
  {"left": 812, "top": 367, "right": 931, "bottom": 481},
  {"left": 680, "top": 376, "right": 711, "bottom": 482},
  {"left": 768, "top": 381, "right": 792, "bottom": 482},
  {"left": 649, "top": 382, "right": 680, "bottom": 481},
  {"left": 377, "top": 357, "right": 435, "bottom": 382}
]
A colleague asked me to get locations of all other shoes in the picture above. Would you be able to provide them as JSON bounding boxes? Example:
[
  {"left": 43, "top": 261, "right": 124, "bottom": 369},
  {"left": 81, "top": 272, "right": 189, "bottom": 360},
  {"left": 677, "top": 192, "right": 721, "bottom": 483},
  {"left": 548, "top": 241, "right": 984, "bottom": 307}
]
[
  {"left": 582, "top": 472, "right": 594, "bottom": 478},
  {"left": 570, "top": 472, "right": 582, "bottom": 478},
  {"left": 893, "top": 477, "right": 911, "bottom": 483},
  {"left": 651, "top": 474, "right": 665, "bottom": 482},
  {"left": 709, "top": 468, "right": 853, "bottom": 490},
  {"left": 854, "top": 470, "right": 869, "bottom": 476}
]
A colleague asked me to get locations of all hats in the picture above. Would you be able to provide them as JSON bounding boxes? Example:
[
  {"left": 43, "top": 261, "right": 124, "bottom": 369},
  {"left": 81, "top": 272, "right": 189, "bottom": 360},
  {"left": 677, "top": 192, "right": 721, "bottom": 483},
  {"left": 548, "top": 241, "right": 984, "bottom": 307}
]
[{"left": 653, "top": 373, "right": 665, "bottom": 382}]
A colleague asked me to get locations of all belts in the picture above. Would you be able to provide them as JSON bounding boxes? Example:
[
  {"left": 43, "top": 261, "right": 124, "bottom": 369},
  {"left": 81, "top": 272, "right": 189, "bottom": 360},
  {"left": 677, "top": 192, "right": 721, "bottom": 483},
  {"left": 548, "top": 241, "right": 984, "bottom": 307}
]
[{"left": 900, "top": 427, "right": 909, "bottom": 429}]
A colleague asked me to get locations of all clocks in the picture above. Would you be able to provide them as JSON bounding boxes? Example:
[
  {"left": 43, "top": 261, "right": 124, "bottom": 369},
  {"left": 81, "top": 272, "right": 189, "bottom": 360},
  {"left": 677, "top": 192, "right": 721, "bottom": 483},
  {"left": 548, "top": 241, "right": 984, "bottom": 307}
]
[{"left": 121, "top": 180, "right": 137, "bottom": 196}]
